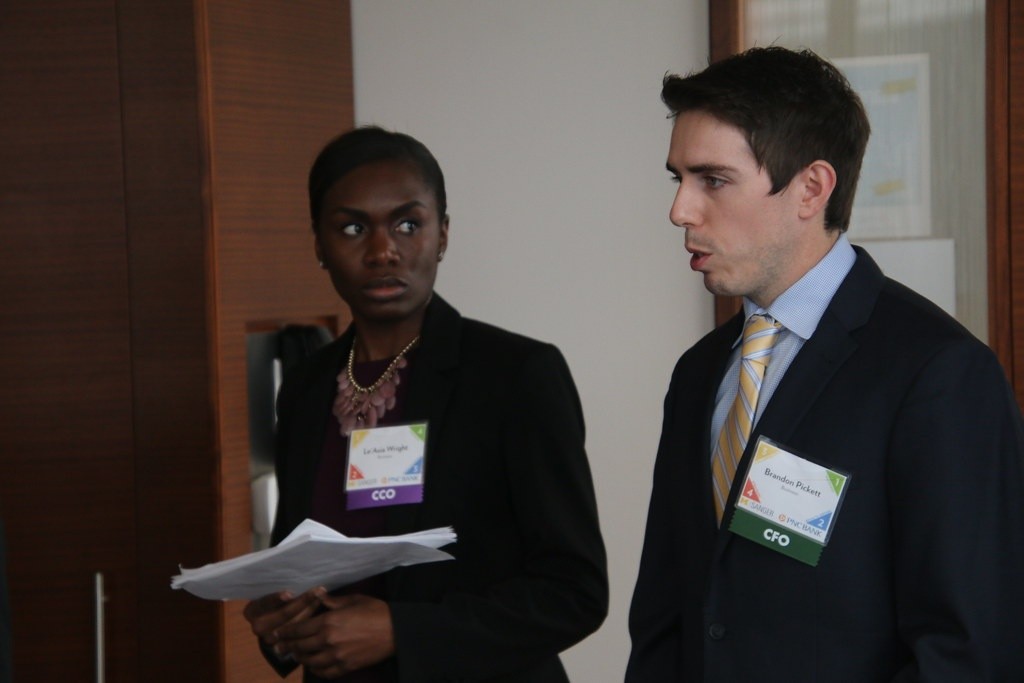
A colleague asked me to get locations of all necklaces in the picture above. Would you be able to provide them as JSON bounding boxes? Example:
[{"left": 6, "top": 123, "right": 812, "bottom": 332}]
[{"left": 332, "top": 335, "right": 421, "bottom": 436}]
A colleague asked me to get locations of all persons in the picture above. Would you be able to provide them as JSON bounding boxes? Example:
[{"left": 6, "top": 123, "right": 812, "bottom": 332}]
[
  {"left": 244, "top": 127, "right": 610, "bottom": 683},
  {"left": 622, "top": 47, "right": 1024, "bottom": 683}
]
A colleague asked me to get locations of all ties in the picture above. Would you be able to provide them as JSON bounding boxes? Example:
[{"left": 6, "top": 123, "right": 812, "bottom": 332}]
[{"left": 710, "top": 312, "right": 787, "bottom": 529}]
